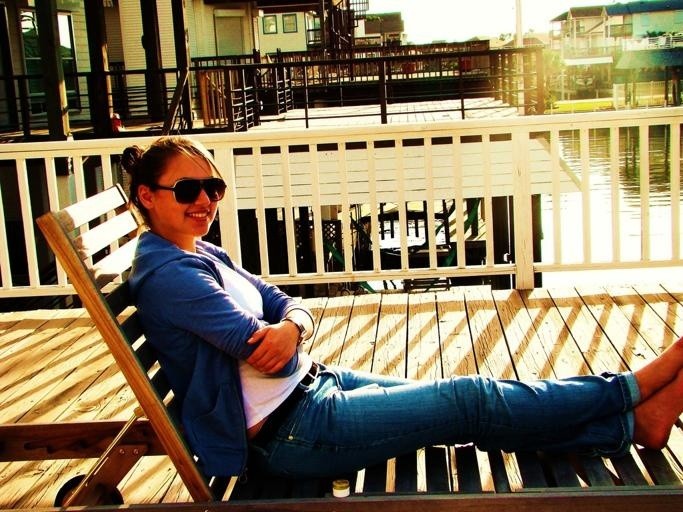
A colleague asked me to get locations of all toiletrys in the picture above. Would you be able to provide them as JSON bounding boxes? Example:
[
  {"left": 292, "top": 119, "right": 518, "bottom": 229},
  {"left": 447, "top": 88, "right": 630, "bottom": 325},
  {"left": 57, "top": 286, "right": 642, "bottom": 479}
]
[{"left": 332, "top": 479, "right": 351, "bottom": 498}]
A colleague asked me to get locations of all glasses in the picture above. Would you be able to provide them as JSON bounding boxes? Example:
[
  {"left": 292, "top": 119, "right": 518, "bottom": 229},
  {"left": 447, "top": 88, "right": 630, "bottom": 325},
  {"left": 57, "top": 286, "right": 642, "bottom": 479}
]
[{"left": 155, "top": 178, "right": 227, "bottom": 203}]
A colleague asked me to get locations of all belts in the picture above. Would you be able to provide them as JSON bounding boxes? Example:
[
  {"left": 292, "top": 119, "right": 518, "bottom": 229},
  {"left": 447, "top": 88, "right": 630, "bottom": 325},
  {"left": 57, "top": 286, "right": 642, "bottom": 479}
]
[{"left": 259, "top": 361, "right": 321, "bottom": 449}]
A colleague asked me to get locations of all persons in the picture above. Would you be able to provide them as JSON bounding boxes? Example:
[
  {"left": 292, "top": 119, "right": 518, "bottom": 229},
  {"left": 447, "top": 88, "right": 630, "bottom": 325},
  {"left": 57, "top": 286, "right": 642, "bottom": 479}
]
[{"left": 121, "top": 131, "right": 683, "bottom": 481}]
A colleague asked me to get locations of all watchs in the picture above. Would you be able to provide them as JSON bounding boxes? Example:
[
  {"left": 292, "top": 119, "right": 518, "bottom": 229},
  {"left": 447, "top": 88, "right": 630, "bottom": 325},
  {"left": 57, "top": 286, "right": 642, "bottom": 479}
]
[{"left": 280, "top": 316, "right": 308, "bottom": 345}]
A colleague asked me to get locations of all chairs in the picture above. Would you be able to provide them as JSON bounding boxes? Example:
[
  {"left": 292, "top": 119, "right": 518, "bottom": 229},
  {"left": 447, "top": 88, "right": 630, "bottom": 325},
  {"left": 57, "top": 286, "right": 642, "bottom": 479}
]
[{"left": 1, "top": 183, "right": 683, "bottom": 512}]
